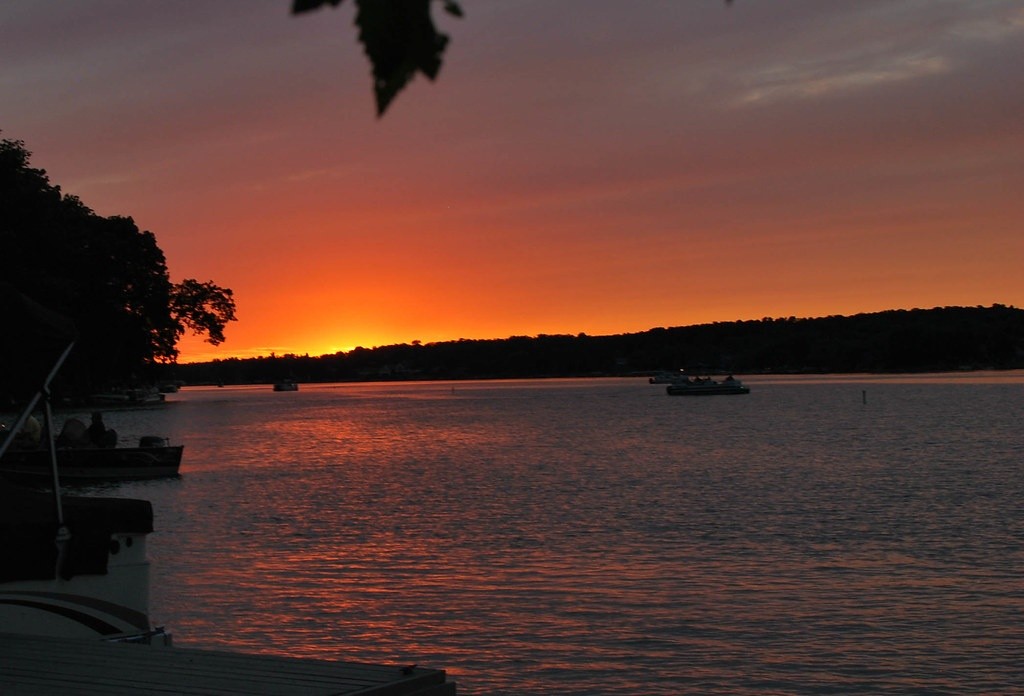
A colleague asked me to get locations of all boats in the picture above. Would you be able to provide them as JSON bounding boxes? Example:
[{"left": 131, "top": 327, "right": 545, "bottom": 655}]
[
  {"left": 650, "top": 375, "right": 691, "bottom": 383},
  {"left": 84, "top": 393, "right": 165, "bottom": 405},
  {"left": 665, "top": 378, "right": 749, "bottom": 398},
  {"left": 1, "top": 431, "right": 183, "bottom": 479},
  {"left": 274, "top": 382, "right": 297, "bottom": 391},
  {"left": 0, "top": 487, "right": 154, "bottom": 533}
]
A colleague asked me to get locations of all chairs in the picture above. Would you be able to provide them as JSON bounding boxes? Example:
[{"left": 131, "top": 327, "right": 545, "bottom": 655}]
[{"left": 96, "top": 429, "right": 117, "bottom": 448}]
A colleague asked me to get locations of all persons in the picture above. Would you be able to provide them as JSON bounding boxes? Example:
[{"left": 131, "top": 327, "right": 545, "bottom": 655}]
[{"left": 8, "top": 405, "right": 107, "bottom": 450}]
